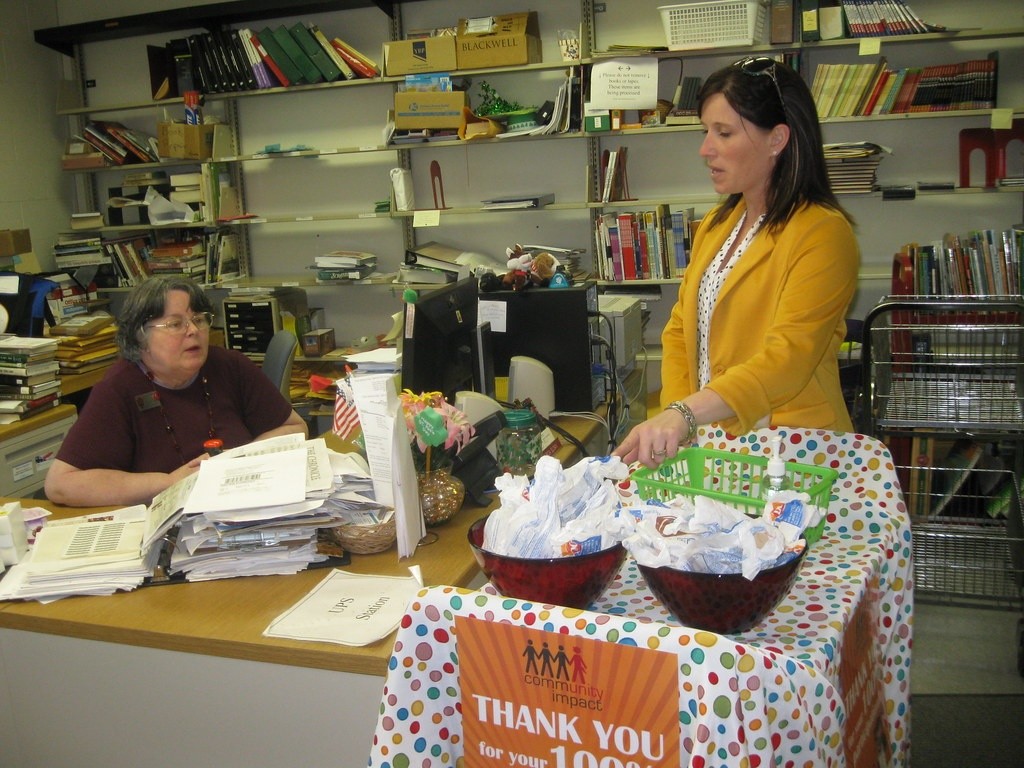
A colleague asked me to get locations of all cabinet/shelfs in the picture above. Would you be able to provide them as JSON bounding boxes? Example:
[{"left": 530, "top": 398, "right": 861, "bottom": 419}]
[{"left": 34, "top": 0, "right": 1024, "bottom": 524}]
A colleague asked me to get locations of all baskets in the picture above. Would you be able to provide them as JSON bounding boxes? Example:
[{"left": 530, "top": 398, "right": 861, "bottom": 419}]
[
  {"left": 629, "top": 449, "right": 838, "bottom": 546},
  {"left": 331, "top": 506, "right": 396, "bottom": 553},
  {"left": 657, "top": 0, "right": 768, "bottom": 52}
]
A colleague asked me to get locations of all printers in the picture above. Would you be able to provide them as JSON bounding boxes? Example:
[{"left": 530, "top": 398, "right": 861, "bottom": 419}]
[{"left": 598, "top": 296, "right": 642, "bottom": 390}]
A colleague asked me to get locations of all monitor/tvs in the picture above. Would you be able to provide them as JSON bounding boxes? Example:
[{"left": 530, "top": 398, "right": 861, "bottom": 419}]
[{"left": 400, "top": 277, "right": 481, "bottom": 406}]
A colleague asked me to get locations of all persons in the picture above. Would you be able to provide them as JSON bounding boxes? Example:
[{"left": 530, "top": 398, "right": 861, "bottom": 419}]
[
  {"left": 44, "top": 274, "right": 310, "bottom": 507},
  {"left": 610, "top": 55, "right": 860, "bottom": 471}
]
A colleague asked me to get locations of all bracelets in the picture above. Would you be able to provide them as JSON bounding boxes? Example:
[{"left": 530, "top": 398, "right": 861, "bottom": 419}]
[{"left": 664, "top": 400, "right": 697, "bottom": 447}]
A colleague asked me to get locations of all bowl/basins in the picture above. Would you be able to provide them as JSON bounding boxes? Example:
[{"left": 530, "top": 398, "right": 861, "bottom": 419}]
[
  {"left": 636, "top": 514, "right": 808, "bottom": 633},
  {"left": 467, "top": 514, "right": 629, "bottom": 610}
]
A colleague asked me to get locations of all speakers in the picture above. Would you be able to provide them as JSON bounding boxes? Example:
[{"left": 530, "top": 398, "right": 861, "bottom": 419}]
[
  {"left": 453, "top": 391, "right": 505, "bottom": 462},
  {"left": 506, "top": 356, "right": 556, "bottom": 422}
]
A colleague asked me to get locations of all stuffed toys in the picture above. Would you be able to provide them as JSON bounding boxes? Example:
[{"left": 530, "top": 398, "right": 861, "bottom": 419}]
[{"left": 502, "top": 243, "right": 561, "bottom": 290}]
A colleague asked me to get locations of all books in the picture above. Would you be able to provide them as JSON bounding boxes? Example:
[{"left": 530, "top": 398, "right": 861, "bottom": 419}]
[
  {"left": 70, "top": 212, "right": 106, "bottom": 230},
  {"left": 145, "top": 19, "right": 381, "bottom": 99},
  {"left": 876, "top": 427, "right": 1024, "bottom": 525},
  {"left": 595, "top": 205, "right": 703, "bottom": 281},
  {"left": 397, "top": 240, "right": 590, "bottom": 284},
  {"left": 0, "top": 163, "right": 240, "bottom": 425},
  {"left": 774, "top": 48, "right": 801, "bottom": 75},
  {"left": 289, "top": 360, "right": 358, "bottom": 416},
  {"left": 304, "top": 251, "right": 378, "bottom": 285},
  {"left": 0, "top": 373, "right": 427, "bottom": 604},
  {"left": 822, "top": 140, "right": 893, "bottom": 195},
  {"left": 1001, "top": 177, "right": 1024, "bottom": 186},
  {"left": 62, "top": 120, "right": 179, "bottom": 170},
  {"left": 810, "top": 50, "right": 999, "bottom": 117},
  {"left": 794, "top": 0, "right": 983, "bottom": 43},
  {"left": 883, "top": 181, "right": 954, "bottom": 198},
  {"left": 900, "top": 224, "right": 1024, "bottom": 314}
]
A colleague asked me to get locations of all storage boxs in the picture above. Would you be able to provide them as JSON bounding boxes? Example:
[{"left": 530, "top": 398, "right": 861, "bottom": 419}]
[
  {"left": 155, "top": 120, "right": 218, "bottom": 160},
  {"left": 303, "top": 329, "right": 335, "bottom": 357},
  {"left": 0, "top": 229, "right": 42, "bottom": 276},
  {"left": 64, "top": 153, "right": 104, "bottom": 170},
  {"left": 394, "top": 92, "right": 470, "bottom": 130},
  {"left": 383, "top": 27, "right": 458, "bottom": 75},
  {"left": 457, "top": 9, "right": 543, "bottom": 70}
]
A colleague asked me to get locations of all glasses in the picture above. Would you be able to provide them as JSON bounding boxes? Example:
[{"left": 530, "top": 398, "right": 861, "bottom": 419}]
[
  {"left": 733, "top": 57, "right": 788, "bottom": 123},
  {"left": 144, "top": 312, "right": 215, "bottom": 335}
]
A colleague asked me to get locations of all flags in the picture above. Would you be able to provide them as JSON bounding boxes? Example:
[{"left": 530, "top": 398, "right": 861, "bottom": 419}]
[{"left": 332, "top": 375, "right": 360, "bottom": 441}]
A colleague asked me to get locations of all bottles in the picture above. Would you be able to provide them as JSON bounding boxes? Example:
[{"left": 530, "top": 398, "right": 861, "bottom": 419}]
[{"left": 497, "top": 410, "right": 541, "bottom": 479}]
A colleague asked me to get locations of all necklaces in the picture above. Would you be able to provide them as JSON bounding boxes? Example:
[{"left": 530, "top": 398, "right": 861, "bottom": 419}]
[{"left": 146, "top": 368, "right": 217, "bottom": 464}]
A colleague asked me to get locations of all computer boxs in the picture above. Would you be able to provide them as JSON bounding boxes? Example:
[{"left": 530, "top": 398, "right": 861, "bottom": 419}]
[{"left": 482, "top": 281, "right": 606, "bottom": 413}]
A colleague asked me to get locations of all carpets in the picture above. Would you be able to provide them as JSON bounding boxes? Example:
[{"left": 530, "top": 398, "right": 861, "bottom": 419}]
[{"left": 908, "top": 693, "right": 1024, "bottom": 768}]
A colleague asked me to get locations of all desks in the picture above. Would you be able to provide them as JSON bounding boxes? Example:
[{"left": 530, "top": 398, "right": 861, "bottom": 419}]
[
  {"left": 0, "top": 360, "right": 117, "bottom": 500},
  {"left": 0, "top": 369, "right": 648, "bottom": 768}
]
